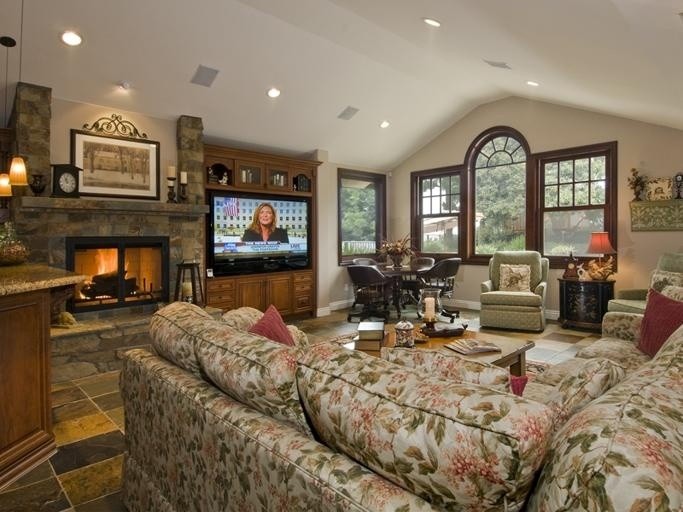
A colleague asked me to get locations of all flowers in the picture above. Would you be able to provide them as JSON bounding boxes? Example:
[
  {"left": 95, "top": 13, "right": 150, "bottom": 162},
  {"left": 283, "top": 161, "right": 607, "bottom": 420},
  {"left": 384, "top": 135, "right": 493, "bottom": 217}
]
[
  {"left": 373, "top": 233, "right": 418, "bottom": 264},
  {"left": 625, "top": 168, "right": 648, "bottom": 194}
]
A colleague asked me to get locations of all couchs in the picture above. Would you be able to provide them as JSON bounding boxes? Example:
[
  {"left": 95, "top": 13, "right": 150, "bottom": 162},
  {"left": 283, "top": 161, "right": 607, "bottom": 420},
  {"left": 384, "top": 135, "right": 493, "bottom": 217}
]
[
  {"left": 347, "top": 266, "right": 402, "bottom": 323},
  {"left": 353, "top": 258, "right": 401, "bottom": 281},
  {"left": 121, "top": 302, "right": 683, "bottom": 512},
  {"left": 418, "top": 256, "right": 462, "bottom": 323},
  {"left": 481, "top": 252, "right": 549, "bottom": 333},
  {"left": 608, "top": 254, "right": 682, "bottom": 313},
  {"left": 411, "top": 256, "right": 434, "bottom": 269}
]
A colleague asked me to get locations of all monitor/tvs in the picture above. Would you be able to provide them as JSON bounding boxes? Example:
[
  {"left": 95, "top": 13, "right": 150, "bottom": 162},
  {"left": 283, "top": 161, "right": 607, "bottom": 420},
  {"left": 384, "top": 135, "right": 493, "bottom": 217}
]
[{"left": 206, "top": 187, "right": 312, "bottom": 277}]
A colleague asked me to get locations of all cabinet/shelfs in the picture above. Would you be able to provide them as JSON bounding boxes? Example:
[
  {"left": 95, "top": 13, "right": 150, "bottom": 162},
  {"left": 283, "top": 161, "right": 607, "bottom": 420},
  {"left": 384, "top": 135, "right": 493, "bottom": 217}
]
[
  {"left": 292, "top": 270, "right": 317, "bottom": 320},
  {"left": 555, "top": 278, "right": 616, "bottom": 334},
  {"left": 0, "top": 264, "right": 93, "bottom": 493},
  {"left": 629, "top": 199, "right": 683, "bottom": 232},
  {"left": 207, "top": 277, "right": 236, "bottom": 311},
  {"left": 236, "top": 271, "right": 294, "bottom": 321},
  {"left": 204, "top": 144, "right": 325, "bottom": 198},
  {"left": 12, "top": 197, "right": 210, "bottom": 323}
]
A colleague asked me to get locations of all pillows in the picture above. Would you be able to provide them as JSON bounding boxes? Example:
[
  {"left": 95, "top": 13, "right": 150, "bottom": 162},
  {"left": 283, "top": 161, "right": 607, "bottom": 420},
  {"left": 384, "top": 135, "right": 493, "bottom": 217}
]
[
  {"left": 646, "top": 269, "right": 682, "bottom": 300},
  {"left": 636, "top": 289, "right": 682, "bottom": 357},
  {"left": 541, "top": 359, "right": 625, "bottom": 437},
  {"left": 498, "top": 262, "right": 532, "bottom": 293},
  {"left": 251, "top": 303, "right": 297, "bottom": 349},
  {"left": 384, "top": 346, "right": 509, "bottom": 392}
]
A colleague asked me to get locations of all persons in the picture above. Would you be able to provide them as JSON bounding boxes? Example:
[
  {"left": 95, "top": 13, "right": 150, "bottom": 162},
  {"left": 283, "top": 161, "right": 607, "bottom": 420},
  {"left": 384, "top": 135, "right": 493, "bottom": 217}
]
[{"left": 240, "top": 204, "right": 289, "bottom": 243}]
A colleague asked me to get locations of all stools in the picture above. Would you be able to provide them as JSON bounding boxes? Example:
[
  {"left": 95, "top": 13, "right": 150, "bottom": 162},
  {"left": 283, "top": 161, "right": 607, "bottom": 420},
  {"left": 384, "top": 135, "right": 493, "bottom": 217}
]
[{"left": 174, "top": 263, "right": 204, "bottom": 304}]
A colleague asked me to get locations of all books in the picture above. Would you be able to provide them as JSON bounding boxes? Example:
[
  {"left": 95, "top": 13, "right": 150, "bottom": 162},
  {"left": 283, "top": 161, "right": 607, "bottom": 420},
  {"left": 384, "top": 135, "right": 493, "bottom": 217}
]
[
  {"left": 357, "top": 321, "right": 385, "bottom": 341},
  {"left": 444, "top": 339, "right": 501, "bottom": 355},
  {"left": 354, "top": 331, "right": 390, "bottom": 351}
]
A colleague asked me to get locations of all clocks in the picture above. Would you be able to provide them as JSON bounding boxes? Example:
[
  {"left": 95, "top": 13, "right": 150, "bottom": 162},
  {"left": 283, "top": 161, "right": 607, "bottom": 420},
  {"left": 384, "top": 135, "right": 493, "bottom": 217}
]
[
  {"left": 50, "top": 163, "right": 82, "bottom": 198},
  {"left": 563, "top": 251, "right": 578, "bottom": 278}
]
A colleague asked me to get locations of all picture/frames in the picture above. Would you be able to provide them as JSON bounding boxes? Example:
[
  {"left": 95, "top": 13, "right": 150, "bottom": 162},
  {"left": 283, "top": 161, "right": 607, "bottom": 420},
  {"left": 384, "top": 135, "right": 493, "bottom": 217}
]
[{"left": 71, "top": 114, "right": 162, "bottom": 200}]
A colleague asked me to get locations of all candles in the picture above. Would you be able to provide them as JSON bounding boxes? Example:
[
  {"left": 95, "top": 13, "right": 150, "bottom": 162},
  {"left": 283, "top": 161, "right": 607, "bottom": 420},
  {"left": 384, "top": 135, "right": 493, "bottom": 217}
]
[
  {"left": 180, "top": 171, "right": 186, "bottom": 184},
  {"left": 168, "top": 181, "right": 173, "bottom": 185},
  {"left": 425, "top": 296, "right": 438, "bottom": 319},
  {"left": 168, "top": 166, "right": 175, "bottom": 179}
]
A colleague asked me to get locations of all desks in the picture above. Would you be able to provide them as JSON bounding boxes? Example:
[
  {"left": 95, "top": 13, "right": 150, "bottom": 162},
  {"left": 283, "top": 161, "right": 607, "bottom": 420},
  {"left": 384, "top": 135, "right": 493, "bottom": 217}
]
[{"left": 378, "top": 264, "right": 432, "bottom": 320}]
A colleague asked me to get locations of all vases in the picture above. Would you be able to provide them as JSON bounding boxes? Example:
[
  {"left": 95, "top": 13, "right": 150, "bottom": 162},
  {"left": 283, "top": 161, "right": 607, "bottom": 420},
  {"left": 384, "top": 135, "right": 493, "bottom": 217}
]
[
  {"left": 632, "top": 191, "right": 642, "bottom": 202},
  {"left": 389, "top": 256, "right": 406, "bottom": 269}
]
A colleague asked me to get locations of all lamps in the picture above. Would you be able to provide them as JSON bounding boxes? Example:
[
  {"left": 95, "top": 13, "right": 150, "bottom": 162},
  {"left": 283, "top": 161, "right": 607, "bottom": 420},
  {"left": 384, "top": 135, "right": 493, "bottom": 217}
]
[
  {"left": 182, "top": 237, "right": 197, "bottom": 262},
  {"left": 587, "top": 232, "right": 616, "bottom": 280},
  {"left": 9, "top": 1, "right": 30, "bottom": 185},
  {"left": 0, "top": 37, "right": 17, "bottom": 198}
]
